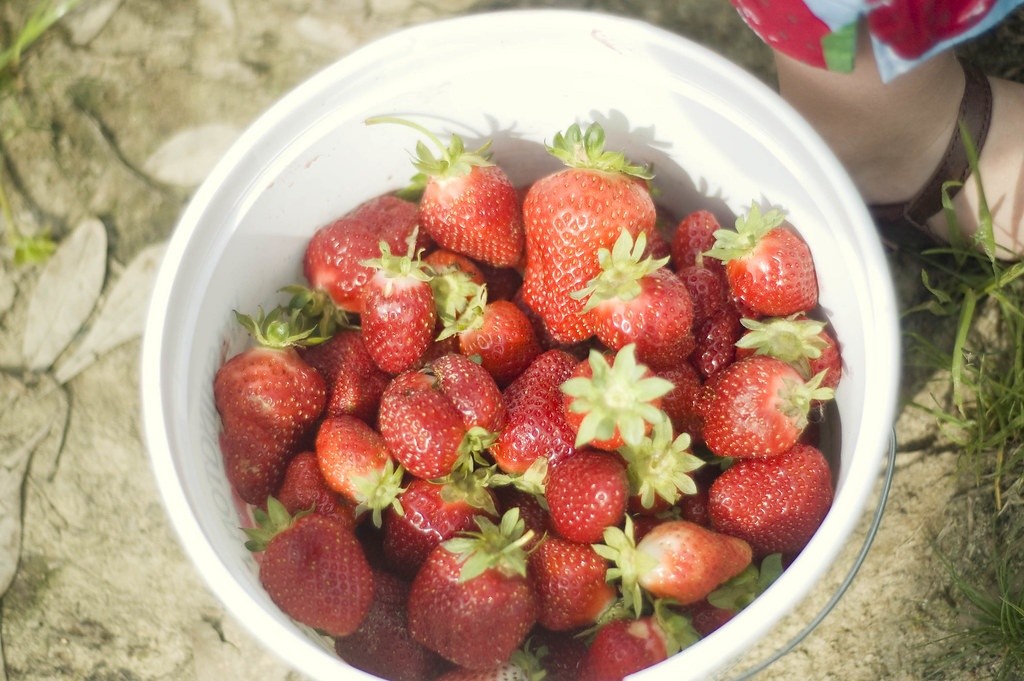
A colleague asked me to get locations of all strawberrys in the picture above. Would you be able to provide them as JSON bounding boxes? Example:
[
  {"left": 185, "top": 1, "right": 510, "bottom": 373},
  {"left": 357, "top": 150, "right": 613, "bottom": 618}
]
[{"left": 209, "top": 116, "right": 843, "bottom": 681}]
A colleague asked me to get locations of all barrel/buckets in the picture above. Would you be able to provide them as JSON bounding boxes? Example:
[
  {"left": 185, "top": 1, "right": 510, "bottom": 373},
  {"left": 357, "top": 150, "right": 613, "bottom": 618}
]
[{"left": 140, "top": 6, "right": 908, "bottom": 681}]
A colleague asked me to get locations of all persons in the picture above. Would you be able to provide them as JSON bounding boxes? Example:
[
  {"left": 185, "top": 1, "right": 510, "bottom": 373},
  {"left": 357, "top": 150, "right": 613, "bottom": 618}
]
[{"left": 728, "top": 0, "right": 1024, "bottom": 261}]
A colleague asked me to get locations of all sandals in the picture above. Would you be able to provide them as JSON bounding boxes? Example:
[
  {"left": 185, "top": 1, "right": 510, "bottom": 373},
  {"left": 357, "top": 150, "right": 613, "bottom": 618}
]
[{"left": 775, "top": 49, "right": 1024, "bottom": 282}]
